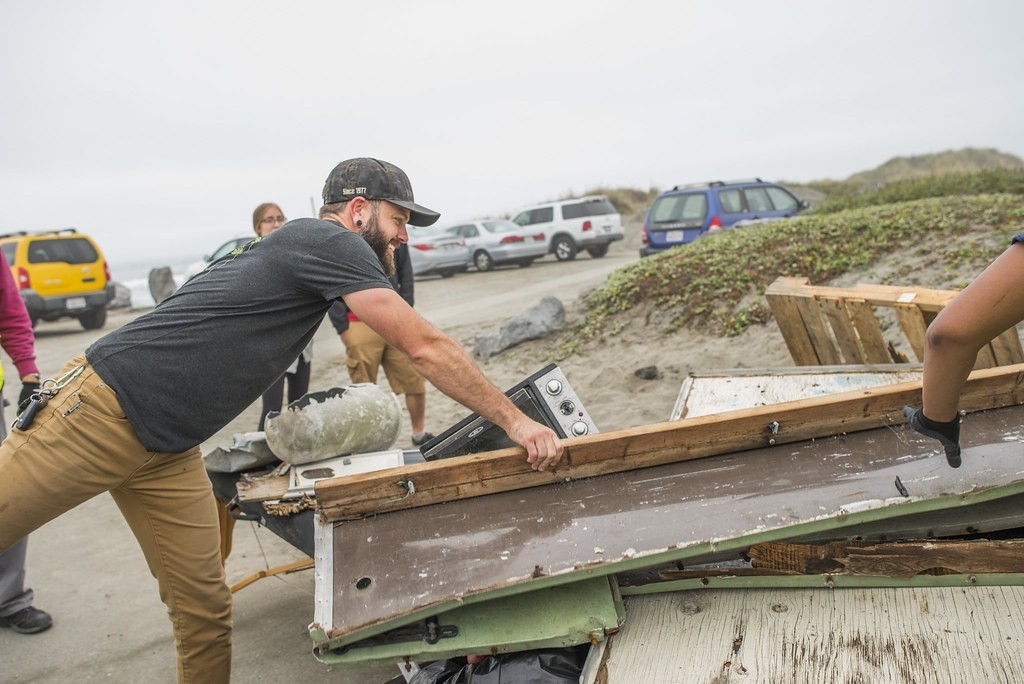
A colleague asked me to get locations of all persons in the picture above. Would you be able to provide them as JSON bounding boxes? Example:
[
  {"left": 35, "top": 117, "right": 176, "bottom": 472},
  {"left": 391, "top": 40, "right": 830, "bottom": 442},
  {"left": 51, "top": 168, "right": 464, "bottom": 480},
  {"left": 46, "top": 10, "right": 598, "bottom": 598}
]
[
  {"left": 0, "top": 157, "right": 565, "bottom": 684},
  {"left": 249, "top": 204, "right": 312, "bottom": 433},
  {"left": 330, "top": 233, "right": 436, "bottom": 446},
  {"left": 0, "top": 247, "right": 52, "bottom": 635},
  {"left": 900, "top": 233, "right": 1024, "bottom": 469}
]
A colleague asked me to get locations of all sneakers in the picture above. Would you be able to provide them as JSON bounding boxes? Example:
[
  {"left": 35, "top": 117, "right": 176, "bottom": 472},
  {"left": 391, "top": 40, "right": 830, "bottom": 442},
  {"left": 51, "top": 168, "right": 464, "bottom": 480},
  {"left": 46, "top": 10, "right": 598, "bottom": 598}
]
[{"left": 0, "top": 606, "right": 53, "bottom": 633}]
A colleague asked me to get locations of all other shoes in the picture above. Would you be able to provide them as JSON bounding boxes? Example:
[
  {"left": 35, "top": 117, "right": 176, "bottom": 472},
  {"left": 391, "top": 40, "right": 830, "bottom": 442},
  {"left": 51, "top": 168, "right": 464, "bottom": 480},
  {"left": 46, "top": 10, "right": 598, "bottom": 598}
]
[{"left": 412, "top": 432, "right": 433, "bottom": 447}]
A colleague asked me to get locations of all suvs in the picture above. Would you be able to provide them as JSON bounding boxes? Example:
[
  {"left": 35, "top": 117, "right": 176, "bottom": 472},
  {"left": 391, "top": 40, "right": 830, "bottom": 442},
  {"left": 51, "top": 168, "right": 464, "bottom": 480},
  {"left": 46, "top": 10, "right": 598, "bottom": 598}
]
[
  {"left": 512, "top": 195, "right": 624, "bottom": 261},
  {"left": 0, "top": 230, "right": 110, "bottom": 330}
]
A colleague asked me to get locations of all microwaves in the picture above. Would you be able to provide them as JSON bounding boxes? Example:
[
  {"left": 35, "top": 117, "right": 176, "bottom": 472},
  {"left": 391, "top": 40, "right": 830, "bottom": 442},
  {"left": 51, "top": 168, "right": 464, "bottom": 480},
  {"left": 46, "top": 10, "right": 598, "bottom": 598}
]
[{"left": 418, "top": 363, "right": 600, "bottom": 463}]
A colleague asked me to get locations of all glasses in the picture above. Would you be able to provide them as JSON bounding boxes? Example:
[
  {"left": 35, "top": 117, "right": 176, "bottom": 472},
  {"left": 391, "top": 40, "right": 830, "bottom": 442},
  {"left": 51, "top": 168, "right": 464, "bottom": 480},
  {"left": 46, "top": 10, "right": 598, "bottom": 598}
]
[{"left": 261, "top": 216, "right": 287, "bottom": 225}]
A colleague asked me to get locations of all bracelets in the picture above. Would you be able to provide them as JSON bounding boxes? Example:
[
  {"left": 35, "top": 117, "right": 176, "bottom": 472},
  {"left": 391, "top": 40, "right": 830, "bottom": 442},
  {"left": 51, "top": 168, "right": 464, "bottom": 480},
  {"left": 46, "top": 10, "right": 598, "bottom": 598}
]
[{"left": 29, "top": 373, "right": 40, "bottom": 379}]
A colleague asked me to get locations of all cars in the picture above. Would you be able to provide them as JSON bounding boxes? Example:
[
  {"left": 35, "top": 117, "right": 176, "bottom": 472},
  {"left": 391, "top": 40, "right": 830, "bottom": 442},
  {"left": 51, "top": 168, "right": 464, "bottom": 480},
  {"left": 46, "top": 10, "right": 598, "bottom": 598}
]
[
  {"left": 639, "top": 178, "right": 809, "bottom": 257},
  {"left": 187, "top": 232, "right": 257, "bottom": 280},
  {"left": 446, "top": 219, "right": 552, "bottom": 269},
  {"left": 407, "top": 227, "right": 469, "bottom": 278}
]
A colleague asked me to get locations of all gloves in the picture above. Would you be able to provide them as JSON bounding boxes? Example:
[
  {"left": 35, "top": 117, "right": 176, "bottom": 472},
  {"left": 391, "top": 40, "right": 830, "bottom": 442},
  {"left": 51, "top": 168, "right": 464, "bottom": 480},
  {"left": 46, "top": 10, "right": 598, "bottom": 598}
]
[
  {"left": 18, "top": 381, "right": 40, "bottom": 412},
  {"left": 902, "top": 406, "right": 962, "bottom": 469}
]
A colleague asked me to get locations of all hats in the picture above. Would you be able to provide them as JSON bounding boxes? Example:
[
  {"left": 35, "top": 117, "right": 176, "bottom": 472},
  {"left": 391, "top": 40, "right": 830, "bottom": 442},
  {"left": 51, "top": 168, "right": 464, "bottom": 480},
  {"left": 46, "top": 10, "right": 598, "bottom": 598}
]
[{"left": 321, "top": 157, "right": 440, "bottom": 227}]
